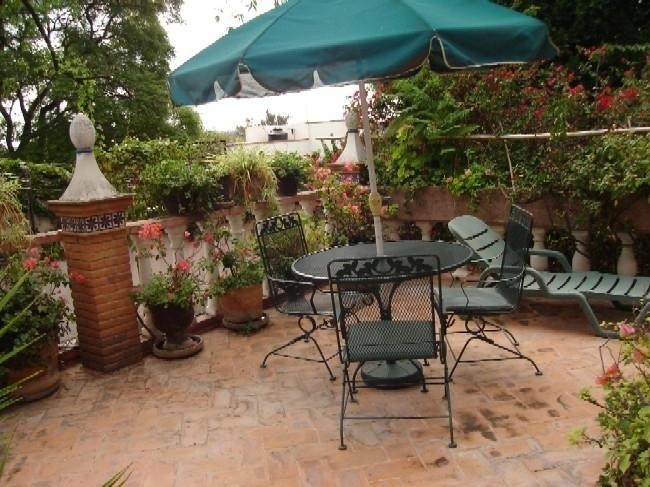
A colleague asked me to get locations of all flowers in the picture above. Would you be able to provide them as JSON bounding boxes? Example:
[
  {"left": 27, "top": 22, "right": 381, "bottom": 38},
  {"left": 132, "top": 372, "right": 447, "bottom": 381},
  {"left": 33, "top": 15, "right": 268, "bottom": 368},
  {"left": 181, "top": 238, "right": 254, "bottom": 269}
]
[
  {"left": 131, "top": 221, "right": 203, "bottom": 302},
  {"left": 4, "top": 247, "right": 84, "bottom": 322},
  {"left": 189, "top": 218, "right": 268, "bottom": 287}
]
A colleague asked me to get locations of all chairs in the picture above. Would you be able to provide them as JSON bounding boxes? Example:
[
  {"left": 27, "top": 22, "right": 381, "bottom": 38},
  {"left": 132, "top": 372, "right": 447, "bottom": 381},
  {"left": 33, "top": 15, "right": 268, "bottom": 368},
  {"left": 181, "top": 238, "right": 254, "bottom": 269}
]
[
  {"left": 446, "top": 212, "right": 650, "bottom": 338},
  {"left": 254, "top": 209, "right": 377, "bottom": 382},
  {"left": 325, "top": 252, "right": 460, "bottom": 451},
  {"left": 422, "top": 202, "right": 545, "bottom": 400}
]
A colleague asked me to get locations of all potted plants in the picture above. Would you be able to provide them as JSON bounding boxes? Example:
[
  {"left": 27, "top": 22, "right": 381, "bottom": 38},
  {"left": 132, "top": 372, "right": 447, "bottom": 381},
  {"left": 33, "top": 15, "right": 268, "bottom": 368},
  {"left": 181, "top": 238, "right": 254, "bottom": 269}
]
[
  {"left": 586, "top": 224, "right": 627, "bottom": 275},
  {"left": 627, "top": 221, "right": 650, "bottom": 277},
  {"left": 544, "top": 229, "right": 575, "bottom": 273},
  {"left": 146, "top": 152, "right": 308, "bottom": 214}
]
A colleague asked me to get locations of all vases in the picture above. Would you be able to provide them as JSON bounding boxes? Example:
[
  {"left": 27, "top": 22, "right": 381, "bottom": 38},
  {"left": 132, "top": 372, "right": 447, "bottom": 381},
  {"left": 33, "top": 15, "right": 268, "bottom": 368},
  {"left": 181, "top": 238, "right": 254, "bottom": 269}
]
[
  {"left": 148, "top": 293, "right": 205, "bottom": 359},
  {"left": 218, "top": 277, "right": 268, "bottom": 333},
  {"left": 1, "top": 325, "right": 64, "bottom": 408}
]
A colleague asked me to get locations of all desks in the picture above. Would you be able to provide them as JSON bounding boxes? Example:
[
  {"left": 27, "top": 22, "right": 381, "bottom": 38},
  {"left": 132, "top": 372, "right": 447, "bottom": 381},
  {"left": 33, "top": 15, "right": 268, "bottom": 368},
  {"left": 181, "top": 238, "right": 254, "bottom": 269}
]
[{"left": 287, "top": 234, "right": 474, "bottom": 390}]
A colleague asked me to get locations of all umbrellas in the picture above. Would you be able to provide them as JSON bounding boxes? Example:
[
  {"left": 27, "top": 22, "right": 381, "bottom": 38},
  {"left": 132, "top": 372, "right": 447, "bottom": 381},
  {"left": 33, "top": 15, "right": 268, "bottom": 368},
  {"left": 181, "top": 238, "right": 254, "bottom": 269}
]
[{"left": 165, "top": 0, "right": 561, "bottom": 258}]
